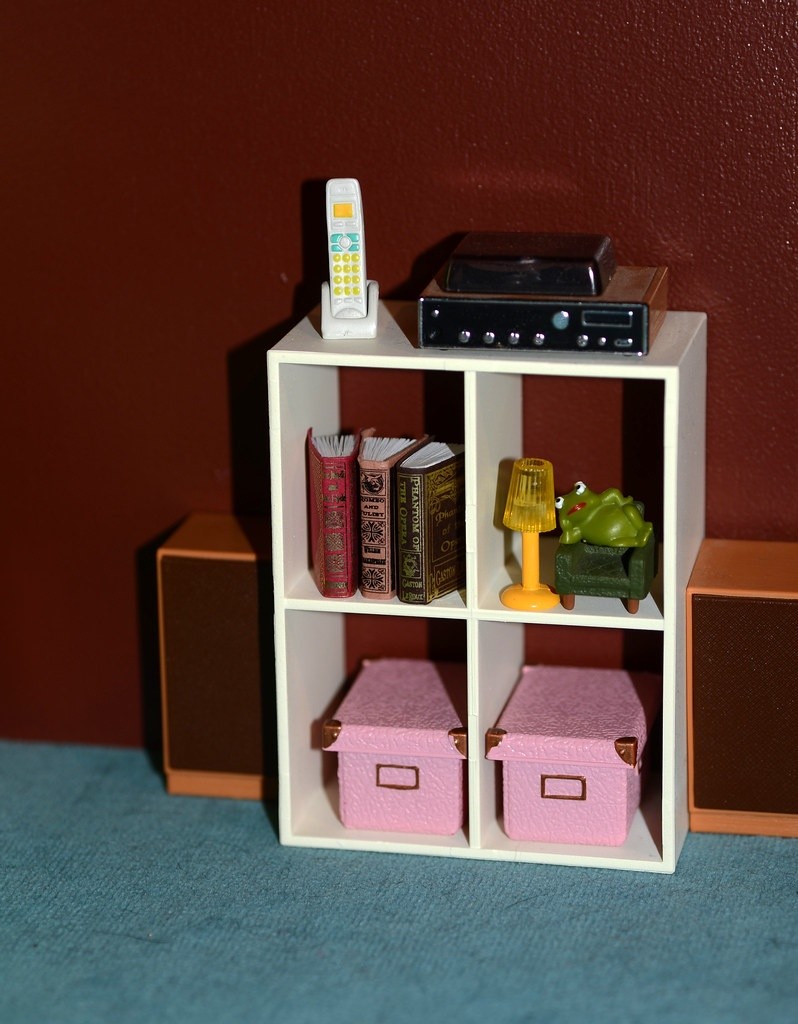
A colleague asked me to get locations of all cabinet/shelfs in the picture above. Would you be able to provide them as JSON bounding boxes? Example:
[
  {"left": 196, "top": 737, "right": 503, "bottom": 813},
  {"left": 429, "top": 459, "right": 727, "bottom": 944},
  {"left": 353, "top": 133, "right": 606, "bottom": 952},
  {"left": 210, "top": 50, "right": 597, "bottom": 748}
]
[
  {"left": 270, "top": 300, "right": 715, "bottom": 883},
  {"left": 151, "top": 512, "right": 279, "bottom": 802},
  {"left": 686, "top": 532, "right": 798, "bottom": 853}
]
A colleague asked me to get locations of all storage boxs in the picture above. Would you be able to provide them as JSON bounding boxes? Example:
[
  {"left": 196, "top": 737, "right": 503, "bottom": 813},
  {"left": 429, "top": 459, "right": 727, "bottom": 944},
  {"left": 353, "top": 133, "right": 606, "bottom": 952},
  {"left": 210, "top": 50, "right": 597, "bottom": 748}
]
[
  {"left": 485, "top": 660, "right": 662, "bottom": 847},
  {"left": 317, "top": 658, "right": 472, "bottom": 838}
]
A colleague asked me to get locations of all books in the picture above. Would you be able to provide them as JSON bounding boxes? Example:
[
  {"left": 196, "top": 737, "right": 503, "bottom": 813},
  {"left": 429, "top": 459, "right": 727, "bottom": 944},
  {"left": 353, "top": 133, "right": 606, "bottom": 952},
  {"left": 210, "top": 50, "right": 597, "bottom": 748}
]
[
  {"left": 393, "top": 435, "right": 465, "bottom": 606},
  {"left": 357, "top": 433, "right": 432, "bottom": 600},
  {"left": 306, "top": 426, "right": 362, "bottom": 598}
]
